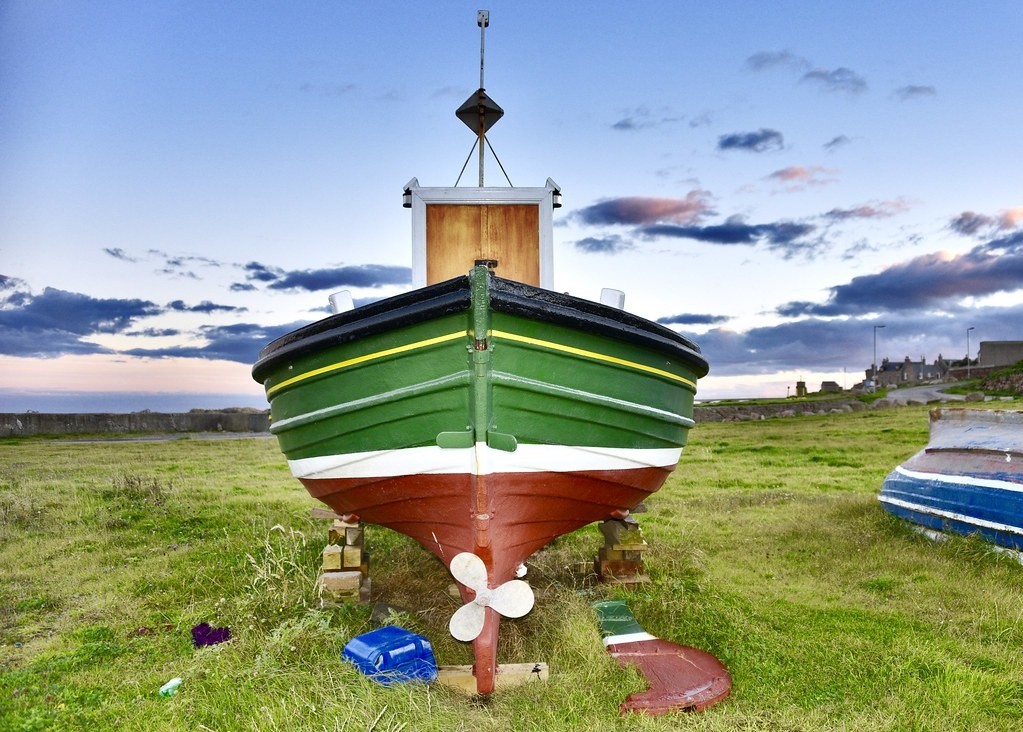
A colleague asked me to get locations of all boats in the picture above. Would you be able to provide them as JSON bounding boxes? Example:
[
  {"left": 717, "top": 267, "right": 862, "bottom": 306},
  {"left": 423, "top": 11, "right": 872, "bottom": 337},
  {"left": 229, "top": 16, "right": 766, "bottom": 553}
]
[
  {"left": 251, "top": 12, "right": 710, "bottom": 698},
  {"left": 874, "top": 408, "right": 1023, "bottom": 566}
]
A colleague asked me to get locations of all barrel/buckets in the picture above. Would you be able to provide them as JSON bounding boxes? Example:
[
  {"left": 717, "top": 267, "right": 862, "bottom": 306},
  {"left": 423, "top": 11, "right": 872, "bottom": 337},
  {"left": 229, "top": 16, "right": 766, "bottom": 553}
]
[{"left": 340, "top": 625, "right": 439, "bottom": 689}]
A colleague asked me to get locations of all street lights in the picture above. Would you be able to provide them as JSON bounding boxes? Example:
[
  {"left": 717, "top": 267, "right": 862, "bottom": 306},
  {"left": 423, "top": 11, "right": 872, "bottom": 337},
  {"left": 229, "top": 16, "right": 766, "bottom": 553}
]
[
  {"left": 967, "top": 327, "right": 974, "bottom": 379},
  {"left": 874, "top": 325, "right": 886, "bottom": 393}
]
